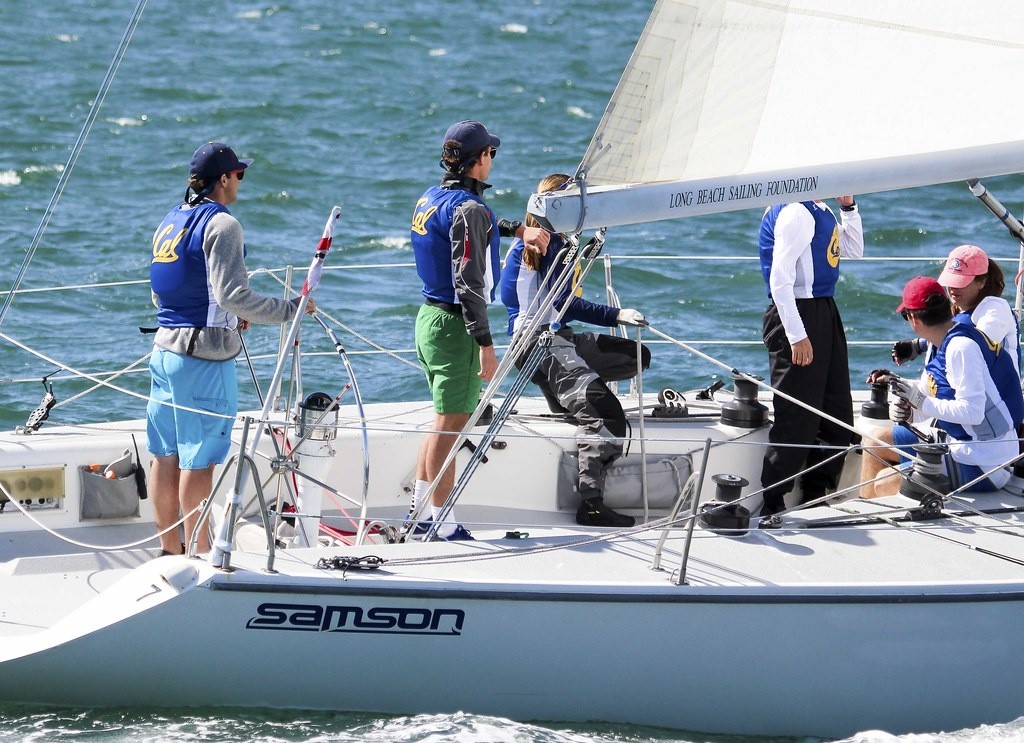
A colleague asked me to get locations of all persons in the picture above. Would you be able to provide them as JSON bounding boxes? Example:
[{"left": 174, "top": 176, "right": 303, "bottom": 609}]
[
  {"left": 759, "top": 195, "right": 865, "bottom": 517},
  {"left": 866, "top": 244, "right": 1022, "bottom": 424},
  {"left": 858, "top": 274, "right": 1024, "bottom": 499},
  {"left": 147, "top": 141, "right": 315, "bottom": 558},
  {"left": 500, "top": 174, "right": 650, "bottom": 527},
  {"left": 400, "top": 117, "right": 551, "bottom": 542}
]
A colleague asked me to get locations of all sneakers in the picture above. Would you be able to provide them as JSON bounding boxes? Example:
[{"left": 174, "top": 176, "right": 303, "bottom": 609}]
[
  {"left": 423, "top": 524, "right": 475, "bottom": 542},
  {"left": 402, "top": 514, "right": 433, "bottom": 539}
]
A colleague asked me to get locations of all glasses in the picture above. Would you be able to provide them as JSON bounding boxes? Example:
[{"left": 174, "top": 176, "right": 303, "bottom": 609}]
[
  {"left": 900, "top": 311, "right": 912, "bottom": 321},
  {"left": 487, "top": 147, "right": 497, "bottom": 159},
  {"left": 237, "top": 171, "right": 245, "bottom": 180}
]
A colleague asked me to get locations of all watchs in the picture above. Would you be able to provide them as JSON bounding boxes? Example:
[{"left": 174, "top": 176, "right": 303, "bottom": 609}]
[{"left": 510, "top": 219, "right": 522, "bottom": 236}]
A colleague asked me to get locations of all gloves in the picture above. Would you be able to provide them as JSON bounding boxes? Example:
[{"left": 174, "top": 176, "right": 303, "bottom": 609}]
[
  {"left": 889, "top": 399, "right": 913, "bottom": 422},
  {"left": 616, "top": 309, "right": 649, "bottom": 327},
  {"left": 889, "top": 377, "right": 926, "bottom": 410}
]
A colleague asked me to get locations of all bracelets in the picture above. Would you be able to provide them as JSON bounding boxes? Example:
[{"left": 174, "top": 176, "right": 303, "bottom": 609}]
[
  {"left": 841, "top": 200, "right": 856, "bottom": 207},
  {"left": 920, "top": 338, "right": 928, "bottom": 352},
  {"left": 912, "top": 338, "right": 922, "bottom": 355},
  {"left": 291, "top": 298, "right": 301, "bottom": 307}
]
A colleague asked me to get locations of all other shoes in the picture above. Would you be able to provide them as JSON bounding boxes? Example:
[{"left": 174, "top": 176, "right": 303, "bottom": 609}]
[
  {"left": 576, "top": 498, "right": 635, "bottom": 526},
  {"left": 799, "top": 499, "right": 830, "bottom": 509},
  {"left": 539, "top": 379, "right": 566, "bottom": 416},
  {"left": 159, "top": 542, "right": 185, "bottom": 557},
  {"left": 759, "top": 504, "right": 786, "bottom": 516}
]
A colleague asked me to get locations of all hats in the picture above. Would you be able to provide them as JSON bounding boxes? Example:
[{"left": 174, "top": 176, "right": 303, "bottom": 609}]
[
  {"left": 896, "top": 276, "right": 946, "bottom": 313},
  {"left": 189, "top": 142, "right": 255, "bottom": 183},
  {"left": 442, "top": 120, "right": 501, "bottom": 162},
  {"left": 938, "top": 245, "right": 989, "bottom": 288}
]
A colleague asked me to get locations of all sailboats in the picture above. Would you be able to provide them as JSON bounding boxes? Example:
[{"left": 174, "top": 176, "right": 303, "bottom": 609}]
[{"left": 0, "top": 0, "right": 1023, "bottom": 743}]
